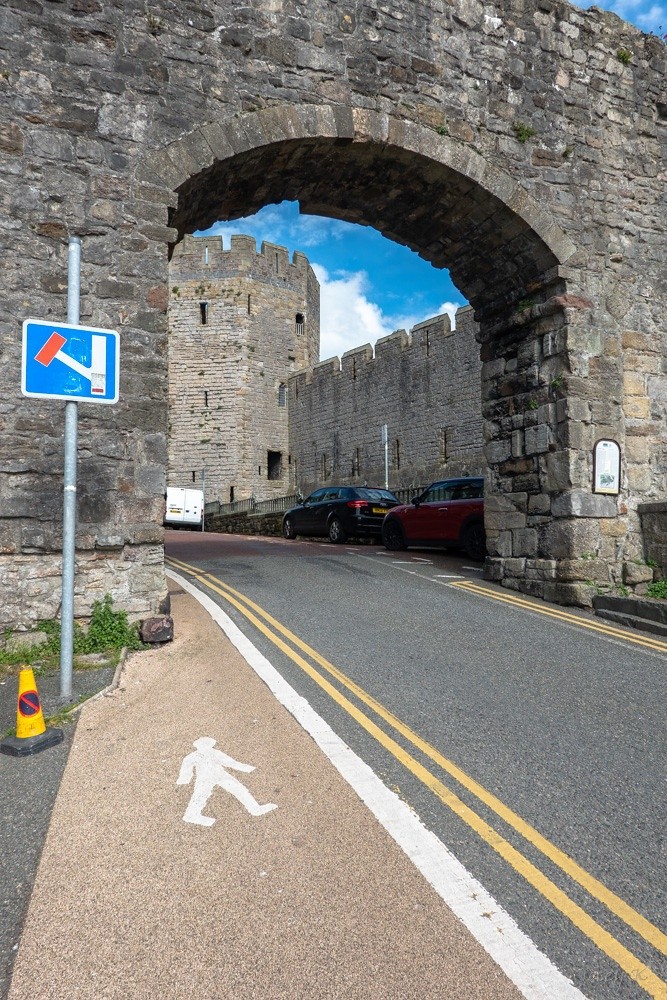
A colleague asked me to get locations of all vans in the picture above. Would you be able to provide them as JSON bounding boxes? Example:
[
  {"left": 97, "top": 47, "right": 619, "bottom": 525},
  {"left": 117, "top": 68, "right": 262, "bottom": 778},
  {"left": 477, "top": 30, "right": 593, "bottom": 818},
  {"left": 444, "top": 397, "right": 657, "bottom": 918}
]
[{"left": 163, "top": 487, "right": 203, "bottom": 531}]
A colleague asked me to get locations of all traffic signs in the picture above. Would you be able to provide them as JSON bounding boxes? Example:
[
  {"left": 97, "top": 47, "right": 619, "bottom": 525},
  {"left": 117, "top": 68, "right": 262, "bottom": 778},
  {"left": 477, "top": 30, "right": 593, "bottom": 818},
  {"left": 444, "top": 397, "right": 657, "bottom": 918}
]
[{"left": 21, "top": 318, "right": 120, "bottom": 405}]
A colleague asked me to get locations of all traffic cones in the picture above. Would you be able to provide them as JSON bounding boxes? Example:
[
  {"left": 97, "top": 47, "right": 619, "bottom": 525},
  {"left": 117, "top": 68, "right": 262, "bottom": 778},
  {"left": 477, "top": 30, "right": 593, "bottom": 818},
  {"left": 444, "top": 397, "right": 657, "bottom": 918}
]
[{"left": 0, "top": 665, "right": 65, "bottom": 759}]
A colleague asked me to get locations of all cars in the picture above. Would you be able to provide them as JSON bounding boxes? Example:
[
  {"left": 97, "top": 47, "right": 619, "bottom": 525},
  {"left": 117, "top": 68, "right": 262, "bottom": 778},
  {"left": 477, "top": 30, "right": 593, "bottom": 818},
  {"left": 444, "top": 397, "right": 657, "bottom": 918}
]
[
  {"left": 382, "top": 475, "right": 487, "bottom": 562},
  {"left": 282, "top": 486, "right": 403, "bottom": 545}
]
